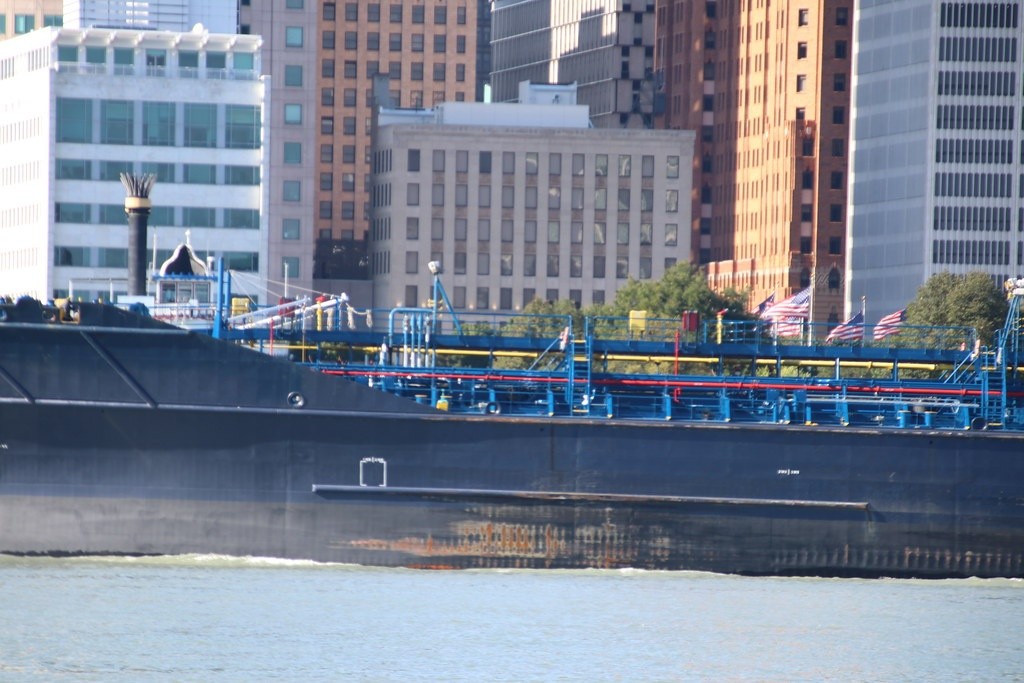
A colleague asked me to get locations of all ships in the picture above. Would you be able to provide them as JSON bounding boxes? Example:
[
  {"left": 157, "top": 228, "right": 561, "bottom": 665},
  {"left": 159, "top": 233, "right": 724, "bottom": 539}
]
[{"left": 0, "top": 298, "right": 1024, "bottom": 583}]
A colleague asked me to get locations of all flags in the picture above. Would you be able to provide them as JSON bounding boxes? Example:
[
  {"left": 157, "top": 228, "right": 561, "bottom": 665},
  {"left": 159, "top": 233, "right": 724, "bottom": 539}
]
[
  {"left": 825, "top": 311, "right": 863, "bottom": 344},
  {"left": 873, "top": 309, "right": 907, "bottom": 341},
  {"left": 751, "top": 286, "right": 811, "bottom": 337}
]
